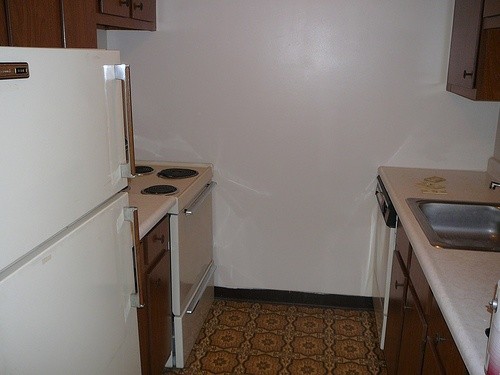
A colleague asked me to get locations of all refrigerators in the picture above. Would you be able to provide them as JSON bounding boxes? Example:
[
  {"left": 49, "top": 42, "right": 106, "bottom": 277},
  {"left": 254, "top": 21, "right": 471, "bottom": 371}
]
[{"left": 1, "top": 47, "right": 145, "bottom": 370}]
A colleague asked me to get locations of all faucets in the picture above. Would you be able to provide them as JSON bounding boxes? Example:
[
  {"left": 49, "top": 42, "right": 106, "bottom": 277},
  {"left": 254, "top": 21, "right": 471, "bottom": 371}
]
[{"left": 490, "top": 182, "right": 500, "bottom": 190}]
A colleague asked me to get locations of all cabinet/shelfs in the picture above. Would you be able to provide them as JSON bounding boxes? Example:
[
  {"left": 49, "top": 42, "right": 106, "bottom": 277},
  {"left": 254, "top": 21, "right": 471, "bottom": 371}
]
[
  {"left": 96, "top": 0, "right": 157, "bottom": 32},
  {"left": 446, "top": 1, "right": 499, "bottom": 101},
  {"left": 384, "top": 216, "right": 432, "bottom": 375},
  {"left": 426, "top": 303, "right": 467, "bottom": 375},
  {"left": 134, "top": 214, "right": 173, "bottom": 375}
]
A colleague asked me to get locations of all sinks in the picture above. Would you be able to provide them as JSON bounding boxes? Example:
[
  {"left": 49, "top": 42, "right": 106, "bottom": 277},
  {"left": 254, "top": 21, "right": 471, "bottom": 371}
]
[{"left": 405, "top": 198, "right": 500, "bottom": 254}]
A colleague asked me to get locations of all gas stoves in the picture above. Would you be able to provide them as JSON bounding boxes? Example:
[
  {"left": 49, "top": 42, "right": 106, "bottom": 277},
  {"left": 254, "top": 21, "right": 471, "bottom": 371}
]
[{"left": 135, "top": 155, "right": 227, "bottom": 209}]
